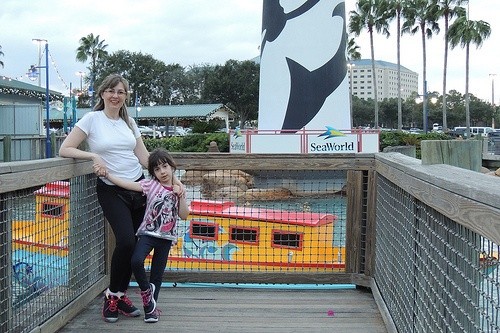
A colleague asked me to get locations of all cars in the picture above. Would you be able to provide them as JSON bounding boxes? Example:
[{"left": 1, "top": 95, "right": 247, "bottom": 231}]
[
  {"left": 410, "top": 127, "right": 420, "bottom": 131},
  {"left": 139, "top": 125, "right": 194, "bottom": 139},
  {"left": 215, "top": 129, "right": 224, "bottom": 133}
]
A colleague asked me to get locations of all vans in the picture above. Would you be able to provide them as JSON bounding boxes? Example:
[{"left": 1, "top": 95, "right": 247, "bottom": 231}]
[
  {"left": 454, "top": 127, "right": 500, "bottom": 136},
  {"left": 432, "top": 123, "right": 442, "bottom": 131}
]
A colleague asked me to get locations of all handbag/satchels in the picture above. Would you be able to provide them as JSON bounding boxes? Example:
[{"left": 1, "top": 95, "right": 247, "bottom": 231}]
[{"left": 131, "top": 193, "right": 147, "bottom": 214}]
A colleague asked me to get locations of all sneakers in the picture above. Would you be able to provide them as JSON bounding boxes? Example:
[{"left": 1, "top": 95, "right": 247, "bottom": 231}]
[
  {"left": 144, "top": 307, "right": 158, "bottom": 322},
  {"left": 119, "top": 290, "right": 141, "bottom": 317},
  {"left": 140, "top": 283, "right": 157, "bottom": 314},
  {"left": 103, "top": 291, "right": 119, "bottom": 321}
]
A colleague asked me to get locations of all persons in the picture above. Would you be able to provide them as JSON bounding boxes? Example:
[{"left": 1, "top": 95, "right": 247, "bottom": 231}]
[
  {"left": 58, "top": 73, "right": 185, "bottom": 322},
  {"left": 92, "top": 148, "right": 189, "bottom": 323}
]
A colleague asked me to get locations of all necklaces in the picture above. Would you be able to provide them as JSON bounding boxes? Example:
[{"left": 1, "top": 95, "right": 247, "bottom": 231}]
[{"left": 104, "top": 110, "right": 120, "bottom": 126}]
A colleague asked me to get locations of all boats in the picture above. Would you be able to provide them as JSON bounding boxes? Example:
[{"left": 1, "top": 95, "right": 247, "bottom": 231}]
[{"left": 11, "top": 180, "right": 357, "bottom": 291}]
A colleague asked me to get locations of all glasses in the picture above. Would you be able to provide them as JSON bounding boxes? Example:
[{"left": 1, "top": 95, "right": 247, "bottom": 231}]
[{"left": 104, "top": 89, "right": 126, "bottom": 96}]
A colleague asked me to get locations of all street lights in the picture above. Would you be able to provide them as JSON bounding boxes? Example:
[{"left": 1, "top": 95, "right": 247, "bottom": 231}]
[
  {"left": 79, "top": 71, "right": 87, "bottom": 92},
  {"left": 489, "top": 73, "right": 498, "bottom": 129},
  {"left": 415, "top": 81, "right": 438, "bottom": 134},
  {"left": 26, "top": 39, "right": 52, "bottom": 161},
  {"left": 135, "top": 90, "right": 141, "bottom": 128},
  {"left": 88, "top": 80, "right": 94, "bottom": 110}
]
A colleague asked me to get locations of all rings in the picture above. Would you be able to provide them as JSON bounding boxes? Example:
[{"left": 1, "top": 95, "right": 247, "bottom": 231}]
[{"left": 182, "top": 192, "right": 184, "bottom": 195}]
